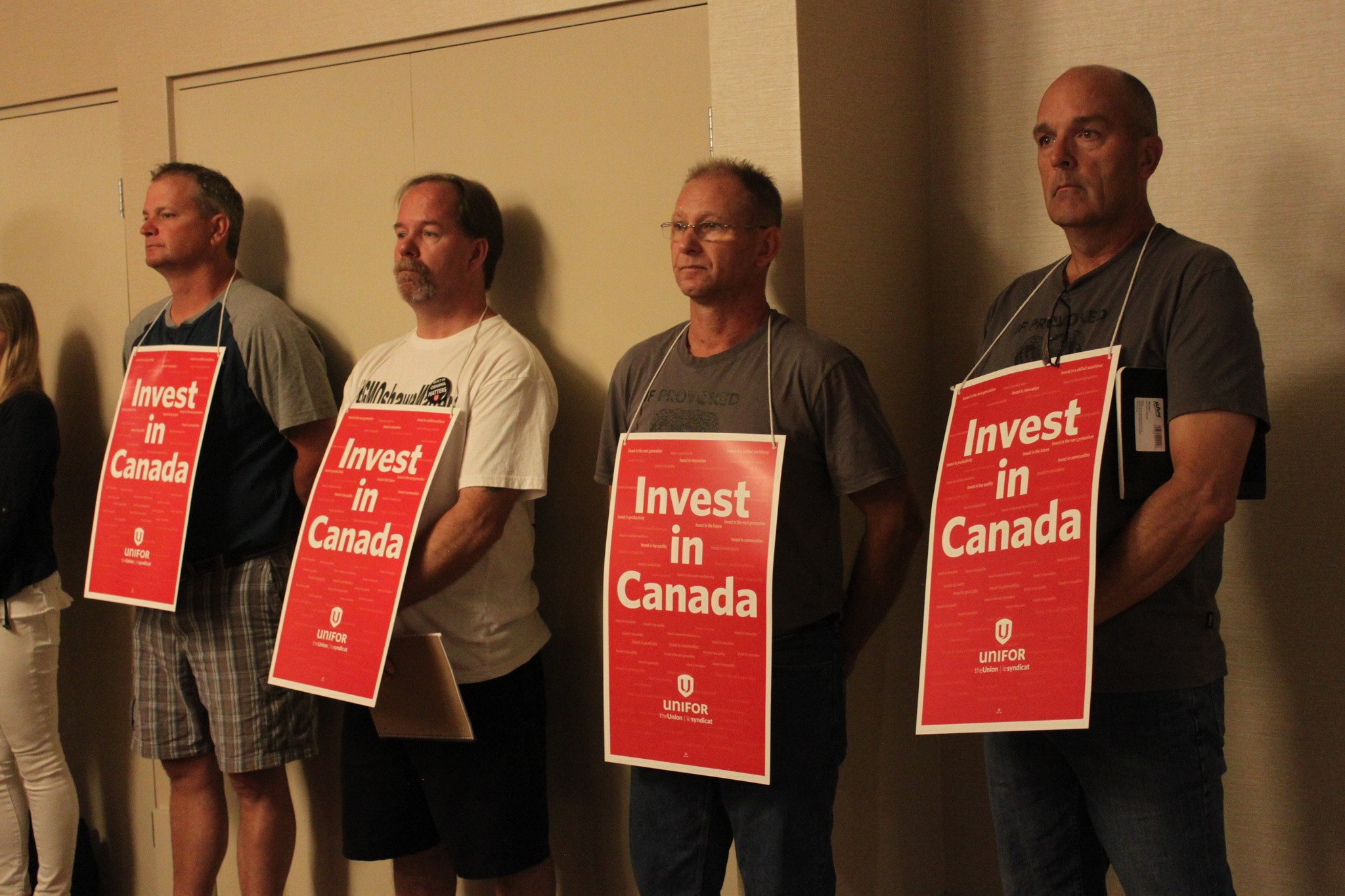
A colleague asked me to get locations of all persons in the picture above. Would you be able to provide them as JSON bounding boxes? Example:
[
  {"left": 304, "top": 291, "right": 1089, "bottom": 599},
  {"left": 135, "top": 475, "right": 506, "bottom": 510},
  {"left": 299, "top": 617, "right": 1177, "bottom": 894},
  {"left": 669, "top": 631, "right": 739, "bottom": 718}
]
[
  {"left": 975, "top": 65, "right": 1273, "bottom": 896},
  {"left": 119, "top": 160, "right": 341, "bottom": 896},
  {"left": 332, "top": 174, "right": 560, "bottom": 896},
  {"left": 594, "top": 158, "right": 923, "bottom": 896},
  {"left": 0, "top": 284, "right": 81, "bottom": 896}
]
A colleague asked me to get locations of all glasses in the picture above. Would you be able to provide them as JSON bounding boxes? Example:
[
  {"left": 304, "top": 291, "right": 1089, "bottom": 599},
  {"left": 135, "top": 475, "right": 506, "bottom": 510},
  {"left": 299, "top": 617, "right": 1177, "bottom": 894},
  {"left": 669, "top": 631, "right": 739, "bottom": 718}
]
[
  {"left": 1041, "top": 287, "right": 1071, "bottom": 366},
  {"left": 660, "top": 221, "right": 769, "bottom": 243}
]
